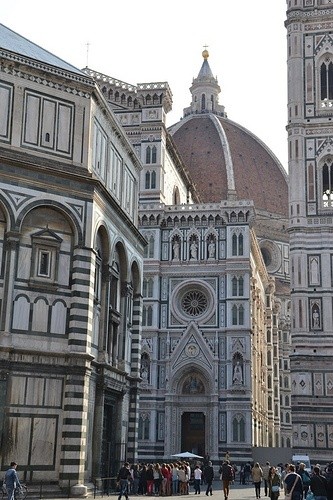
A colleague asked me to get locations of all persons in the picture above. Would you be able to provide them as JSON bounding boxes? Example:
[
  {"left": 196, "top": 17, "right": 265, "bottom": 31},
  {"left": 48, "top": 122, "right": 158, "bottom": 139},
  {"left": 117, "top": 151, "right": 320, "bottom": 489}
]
[
  {"left": 262, "top": 461, "right": 273, "bottom": 498},
  {"left": 200, "top": 463, "right": 207, "bottom": 485},
  {"left": 116, "top": 463, "right": 134, "bottom": 500},
  {"left": 224, "top": 451, "right": 232, "bottom": 465},
  {"left": 193, "top": 466, "right": 202, "bottom": 495},
  {"left": 297, "top": 463, "right": 311, "bottom": 500},
  {"left": 253, "top": 462, "right": 264, "bottom": 500},
  {"left": 243, "top": 460, "right": 252, "bottom": 486},
  {"left": 274, "top": 460, "right": 333, "bottom": 479},
  {"left": 267, "top": 466, "right": 284, "bottom": 500},
  {"left": 323, "top": 465, "right": 333, "bottom": 500},
  {"left": 218, "top": 461, "right": 235, "bottom": 500},
  {"left": 204, "top": 451, "right": 210, "bottom": 465},
  {"left": 310, "top": 467, "right": 327, "bottom": 500},
  {"left": 230, "top": 463, "right": 238, "bottom": 485},
  {"left": 4, "top": 461, "right": 23, "bottom": 500},
  {"left": 282, "top": 465, "right": 304, "bottom": 500},
  {"left": 249, "top": 460, "right": 256, "bottom": 479},
  {"left": 237, "top": 463, "right": 245, "bottom": 485},
  {"left": 130, "top": 460, "right": 192, "bottom": 497},
  {"left": 204, "top": 461, "right": 214, "bottom": 497}
]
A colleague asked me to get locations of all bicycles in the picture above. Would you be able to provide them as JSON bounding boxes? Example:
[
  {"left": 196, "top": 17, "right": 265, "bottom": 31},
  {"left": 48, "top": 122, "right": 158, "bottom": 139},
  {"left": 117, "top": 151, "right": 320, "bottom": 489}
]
[{"left": 0, "top": 477, "right": 29, "bottom": 500}]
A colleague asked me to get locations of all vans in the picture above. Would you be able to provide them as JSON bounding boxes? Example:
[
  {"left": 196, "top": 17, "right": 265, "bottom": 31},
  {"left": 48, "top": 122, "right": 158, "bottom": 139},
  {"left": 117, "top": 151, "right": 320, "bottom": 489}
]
[{"left": 292, "top": 452, "right": 311, "bottom": 477}]
